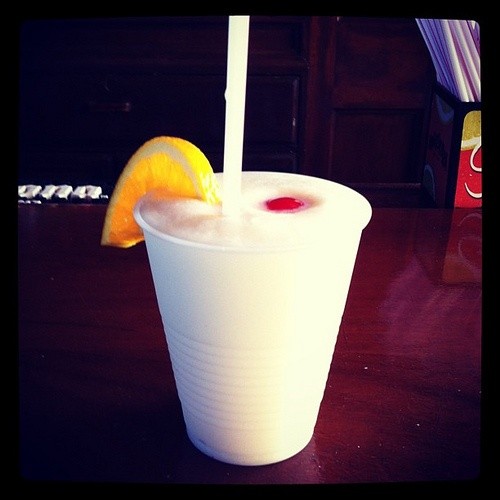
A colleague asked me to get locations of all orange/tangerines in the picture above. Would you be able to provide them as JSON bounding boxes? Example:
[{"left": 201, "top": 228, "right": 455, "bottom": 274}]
[{"left": 99, "top": 136, "right": 220, "bottom": 248}]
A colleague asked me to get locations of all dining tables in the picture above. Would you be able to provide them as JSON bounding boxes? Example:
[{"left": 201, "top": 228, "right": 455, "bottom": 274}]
[{"left": 16, "top": 201, "right": 482, "bottom": 483}]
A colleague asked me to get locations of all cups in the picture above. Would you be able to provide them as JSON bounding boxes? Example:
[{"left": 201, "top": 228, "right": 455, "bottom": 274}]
[{"left": 133, "top": 170, "right": 372, "bottom": 465}]
